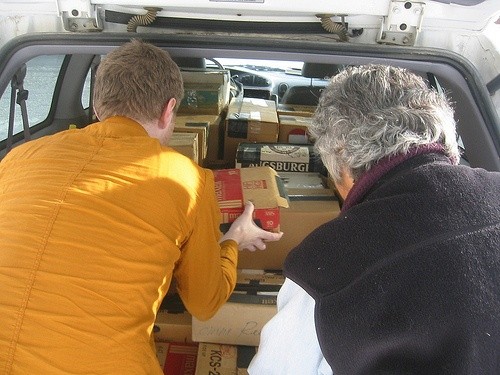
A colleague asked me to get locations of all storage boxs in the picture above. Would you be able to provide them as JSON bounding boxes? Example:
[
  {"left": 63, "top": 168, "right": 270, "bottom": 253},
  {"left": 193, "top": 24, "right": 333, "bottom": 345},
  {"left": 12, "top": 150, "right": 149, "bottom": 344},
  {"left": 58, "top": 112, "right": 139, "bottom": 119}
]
[{"left": 155, "top": 66, "right": 344, "bottom": 375}]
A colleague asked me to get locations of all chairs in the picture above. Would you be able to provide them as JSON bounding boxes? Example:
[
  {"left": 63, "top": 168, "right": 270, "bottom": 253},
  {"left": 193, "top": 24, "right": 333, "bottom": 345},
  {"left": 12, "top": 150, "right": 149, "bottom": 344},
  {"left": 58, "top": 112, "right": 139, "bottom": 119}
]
[{"left": 280, "top": 62, "right": 339, "bottom": 106}]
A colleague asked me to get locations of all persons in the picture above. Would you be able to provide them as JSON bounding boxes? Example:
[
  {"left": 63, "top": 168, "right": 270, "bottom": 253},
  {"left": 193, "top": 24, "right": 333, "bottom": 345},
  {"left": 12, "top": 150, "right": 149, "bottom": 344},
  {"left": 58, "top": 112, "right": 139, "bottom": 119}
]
[
  {"left": 247, "top": 62, "right": 500, "bottom": 375},
  {"left": 0, "top": 37, "right": 283, "bottom": 375}
]
